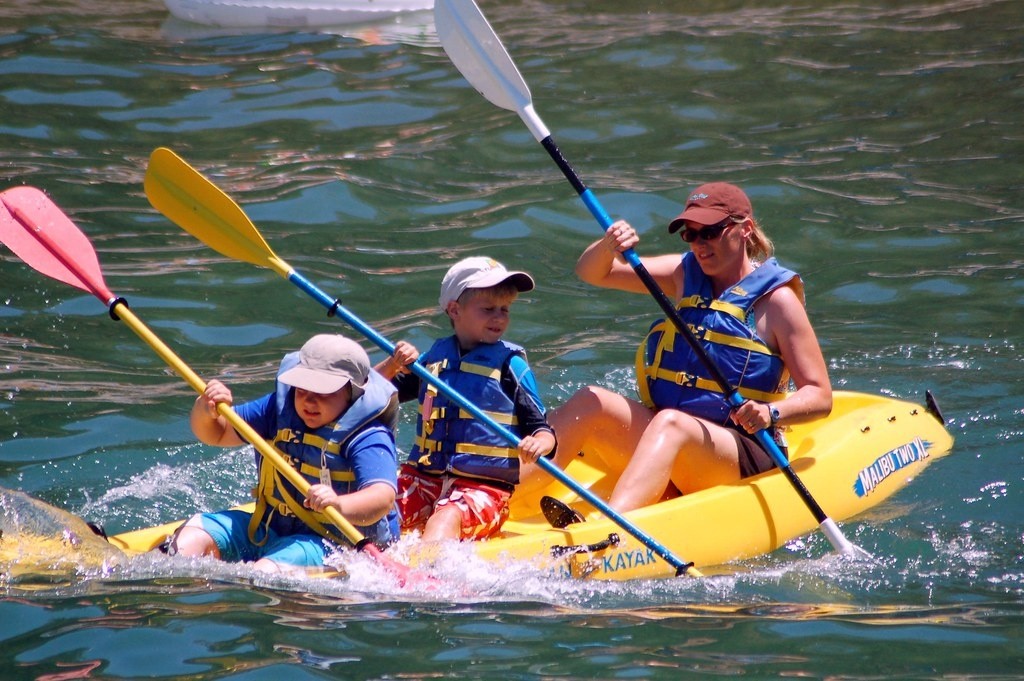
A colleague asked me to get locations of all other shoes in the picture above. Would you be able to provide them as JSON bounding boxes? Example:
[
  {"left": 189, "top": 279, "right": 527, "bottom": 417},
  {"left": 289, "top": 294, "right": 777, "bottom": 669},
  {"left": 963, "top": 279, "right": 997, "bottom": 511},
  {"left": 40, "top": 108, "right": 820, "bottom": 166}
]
[{"left": 540, "top": 496, "right": 587, "bottom": 529}]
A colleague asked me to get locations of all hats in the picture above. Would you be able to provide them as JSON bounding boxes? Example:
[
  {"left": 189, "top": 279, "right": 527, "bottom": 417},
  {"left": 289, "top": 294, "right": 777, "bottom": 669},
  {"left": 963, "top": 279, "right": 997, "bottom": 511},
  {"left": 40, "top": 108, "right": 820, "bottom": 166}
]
[
  {"left": 439, "top": 257, "right": 533, "bottom": 311},
  {"left": 278, "top": 334, "right": 370, "bottom": 393},
  {"left": 668, "top": 183, "right": 752, "bottom": 234}
]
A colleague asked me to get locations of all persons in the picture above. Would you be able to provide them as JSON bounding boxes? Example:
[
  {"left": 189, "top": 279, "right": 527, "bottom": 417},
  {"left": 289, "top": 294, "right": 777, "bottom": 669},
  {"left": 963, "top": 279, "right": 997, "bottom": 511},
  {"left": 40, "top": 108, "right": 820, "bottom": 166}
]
[
  {"left": 374, "top": 256, "right": 558, "bottom": 542},
  {"left": 519, "top": 180, "right": 834, "bottom": 529},
  {"left": 168, "top": 333, "right": 401, "bottom": 571}
]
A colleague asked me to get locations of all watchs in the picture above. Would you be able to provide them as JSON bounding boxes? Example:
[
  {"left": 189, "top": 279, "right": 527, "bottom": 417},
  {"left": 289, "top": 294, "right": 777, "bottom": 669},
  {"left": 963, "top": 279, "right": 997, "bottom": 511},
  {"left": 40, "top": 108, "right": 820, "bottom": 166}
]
[{"left": 767, "top": 403, "right": 780, "bottom": 424}]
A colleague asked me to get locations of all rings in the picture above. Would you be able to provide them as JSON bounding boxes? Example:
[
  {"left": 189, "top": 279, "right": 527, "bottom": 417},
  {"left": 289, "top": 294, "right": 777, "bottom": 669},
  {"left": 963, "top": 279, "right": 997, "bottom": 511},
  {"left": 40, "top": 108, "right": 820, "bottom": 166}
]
[{"left": 618, "top": 228, "right": 623, "bottom": 234}]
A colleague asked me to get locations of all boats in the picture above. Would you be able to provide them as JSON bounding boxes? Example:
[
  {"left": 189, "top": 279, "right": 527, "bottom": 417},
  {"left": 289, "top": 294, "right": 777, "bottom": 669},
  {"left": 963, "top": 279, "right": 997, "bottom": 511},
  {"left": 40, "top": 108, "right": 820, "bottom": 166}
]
[
  {"left": 0, "top": 386, "right": 954, "bottom": 586},
  {"left": 164, "top": 0, "right": 438, "bottom": 30}
]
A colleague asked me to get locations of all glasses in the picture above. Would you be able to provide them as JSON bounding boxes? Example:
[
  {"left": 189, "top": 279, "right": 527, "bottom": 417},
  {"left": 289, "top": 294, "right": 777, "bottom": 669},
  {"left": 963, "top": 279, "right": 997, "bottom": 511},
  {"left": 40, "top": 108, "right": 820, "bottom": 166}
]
[{"left": 678, "top": 222, "right": 738, "bottom": 242}]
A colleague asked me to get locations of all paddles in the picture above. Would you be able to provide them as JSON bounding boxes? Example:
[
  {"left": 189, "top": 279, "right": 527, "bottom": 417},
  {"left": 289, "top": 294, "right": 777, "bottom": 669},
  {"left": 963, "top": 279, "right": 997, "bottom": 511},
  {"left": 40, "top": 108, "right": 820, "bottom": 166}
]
[
  {"left": 0, "top": 186, "right": 450, "bottom": 594},
  {"left": 429, "top": 0, "right": 880, "bottom": 566},
  {"left": 142, "top": 147, "right": 705, "bottom": 576}
]
